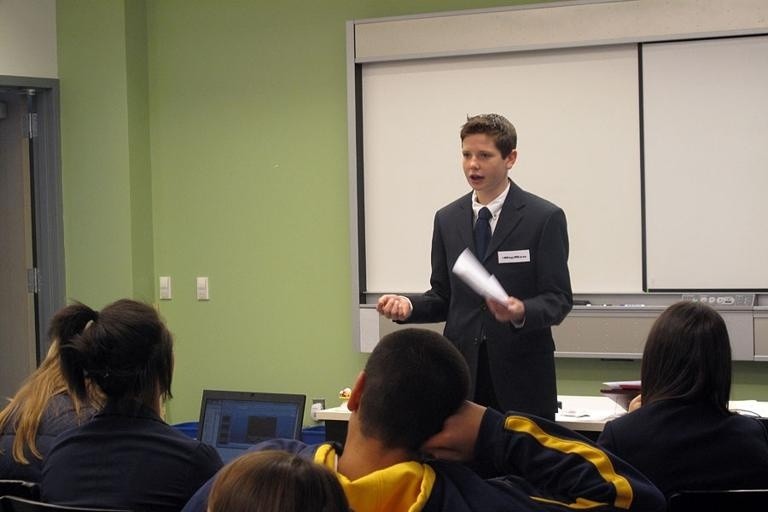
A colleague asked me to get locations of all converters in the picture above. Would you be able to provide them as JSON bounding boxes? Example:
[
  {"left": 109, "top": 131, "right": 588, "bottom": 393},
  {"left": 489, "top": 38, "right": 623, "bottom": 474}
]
[{"left": 310, "top": 403, "right": 323, "bottom": 422}]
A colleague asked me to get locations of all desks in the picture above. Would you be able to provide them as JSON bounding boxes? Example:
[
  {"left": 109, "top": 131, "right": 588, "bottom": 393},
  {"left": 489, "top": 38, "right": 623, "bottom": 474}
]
[{"left": 315, "top": 395, "right": 768, "bottom": 442}]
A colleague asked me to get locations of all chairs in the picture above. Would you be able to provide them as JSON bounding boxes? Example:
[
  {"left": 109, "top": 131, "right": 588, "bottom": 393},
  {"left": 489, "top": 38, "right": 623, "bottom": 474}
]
[
  {"left": 0, "top": 479, "right": 40, "bottom": 501},
  {"left": 0, "top": 494, "right": 131, "bottom": 512},
  {"left": 668, "top": 489, "right": 768, "bottom": 512}
]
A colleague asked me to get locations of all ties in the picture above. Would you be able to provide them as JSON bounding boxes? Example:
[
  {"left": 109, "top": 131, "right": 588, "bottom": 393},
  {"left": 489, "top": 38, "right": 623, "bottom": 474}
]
[{"left": 472, "top": 206, "right": 493, "bottom": 262}]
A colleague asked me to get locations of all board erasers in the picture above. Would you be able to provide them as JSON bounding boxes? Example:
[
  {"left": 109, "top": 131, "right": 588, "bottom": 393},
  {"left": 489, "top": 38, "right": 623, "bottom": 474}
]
[{"left": 572, "top": 300, "right": 591, "bottom": 305}]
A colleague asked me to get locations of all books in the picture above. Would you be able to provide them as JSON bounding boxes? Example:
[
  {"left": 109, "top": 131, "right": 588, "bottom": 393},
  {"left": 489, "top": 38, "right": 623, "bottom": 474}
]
[
  {"left": 450, "top": 248, "right": 511, "bottom": 309},
  {"left": 602, "top": 379, "right": 642, "bottom": 390}
]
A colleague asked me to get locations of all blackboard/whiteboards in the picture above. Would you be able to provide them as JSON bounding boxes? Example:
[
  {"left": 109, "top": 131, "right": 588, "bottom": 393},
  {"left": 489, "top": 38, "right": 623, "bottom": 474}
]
[
  {"left": 347, "top": 0, "right": 768, "bottom": 297},
  {"left": 354, "top": 40, "right": 646, "bottom": 297},
  {"left": 641, "top": 33, "right": 768, "bottom": 296}
]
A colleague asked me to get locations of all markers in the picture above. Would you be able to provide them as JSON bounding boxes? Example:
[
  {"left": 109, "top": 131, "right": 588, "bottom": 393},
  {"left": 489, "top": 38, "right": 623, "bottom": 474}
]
[{"left": 586, "top": 303, "right": 646, "bottom": 307}]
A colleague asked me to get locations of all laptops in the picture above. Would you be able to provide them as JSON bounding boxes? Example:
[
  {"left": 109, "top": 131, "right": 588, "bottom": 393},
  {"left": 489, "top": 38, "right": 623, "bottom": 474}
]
[{"left": 197, "top": 391, "right": 307, "bottom": 470}]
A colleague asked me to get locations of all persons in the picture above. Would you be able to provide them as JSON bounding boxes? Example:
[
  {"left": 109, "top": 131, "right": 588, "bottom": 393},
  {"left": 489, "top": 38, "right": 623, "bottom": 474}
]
[
  {"left": 38, "top": 297, "right": 225, "bottom": 512},
  {"left": 207, "top": 447, "right": 356, "bottom": 512},
  {"left": 376, "top": 113, "right": 575, "bottom": 422},
  {"left": 597, "top": 298, "right": 765, "bottom": 500},
  {"left": 0, "top": 324, "right": 106, "bottom": 484},
  {"left": 181, "top": 327, "right": 669, "bottom": 508}
]
